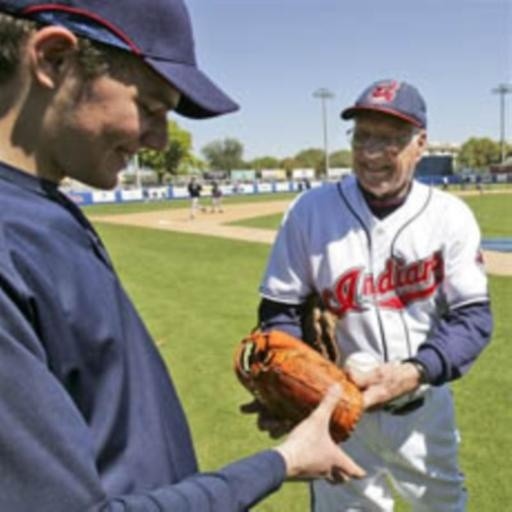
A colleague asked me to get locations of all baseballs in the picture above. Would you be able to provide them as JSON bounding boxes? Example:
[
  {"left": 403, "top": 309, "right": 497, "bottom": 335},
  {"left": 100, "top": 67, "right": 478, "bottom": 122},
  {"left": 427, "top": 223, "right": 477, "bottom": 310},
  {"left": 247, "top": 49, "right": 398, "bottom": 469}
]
[{"left": 344, "top": 351, "right": 382, "bottom": 389}]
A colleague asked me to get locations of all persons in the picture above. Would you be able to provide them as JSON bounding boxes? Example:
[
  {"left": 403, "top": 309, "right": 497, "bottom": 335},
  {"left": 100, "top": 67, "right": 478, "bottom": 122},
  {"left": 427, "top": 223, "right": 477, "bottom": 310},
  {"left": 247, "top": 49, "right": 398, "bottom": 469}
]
[
  {"left": 185, "top": 174, "right": 206, "bottom": 222},
  {"left": 208, "top": 178, "right": 224, "bottom": 212},
  {"left": 239, "top": 81, "right": 492, "bottom": 510},
  {"left": 0, "top": 1, "right": 369, "bottom": 510},
  {"left": 431, "top": 167, "right": 487, "bottom": 194}
]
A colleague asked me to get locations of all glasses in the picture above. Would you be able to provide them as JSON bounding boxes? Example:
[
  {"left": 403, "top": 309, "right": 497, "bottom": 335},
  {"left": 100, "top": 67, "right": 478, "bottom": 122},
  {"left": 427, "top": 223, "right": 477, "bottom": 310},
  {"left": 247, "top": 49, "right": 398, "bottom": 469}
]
[{"left": 345, "top": 127, "right": 418, "bottom": 153}]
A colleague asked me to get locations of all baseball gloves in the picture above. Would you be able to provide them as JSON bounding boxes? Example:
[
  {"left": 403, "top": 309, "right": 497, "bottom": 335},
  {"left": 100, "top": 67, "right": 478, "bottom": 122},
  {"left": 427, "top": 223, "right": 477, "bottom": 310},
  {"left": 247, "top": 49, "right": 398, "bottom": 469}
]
[
  {"left": 234, "top": 328, "right": 364, "bottom": 443},
  {"left": 303, "top": 293, "right": 341, "bottom": 367}
]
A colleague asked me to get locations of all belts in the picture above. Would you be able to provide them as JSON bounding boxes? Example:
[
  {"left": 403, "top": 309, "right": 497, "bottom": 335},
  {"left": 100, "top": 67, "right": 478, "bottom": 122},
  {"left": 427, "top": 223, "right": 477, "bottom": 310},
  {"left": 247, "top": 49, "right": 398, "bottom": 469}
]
[{"left": 381, "top": 398, "right": 425, "bottom": 416}]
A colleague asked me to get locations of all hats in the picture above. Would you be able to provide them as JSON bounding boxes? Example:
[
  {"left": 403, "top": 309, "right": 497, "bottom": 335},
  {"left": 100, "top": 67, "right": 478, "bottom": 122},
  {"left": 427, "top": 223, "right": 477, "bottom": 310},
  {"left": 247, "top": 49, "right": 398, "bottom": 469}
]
[
  {"left": 1, "top": 0, "right": 240, "bottom": 120},
  {"left": 341, "top": 79, "right": 427, "bottom": 129}
]
